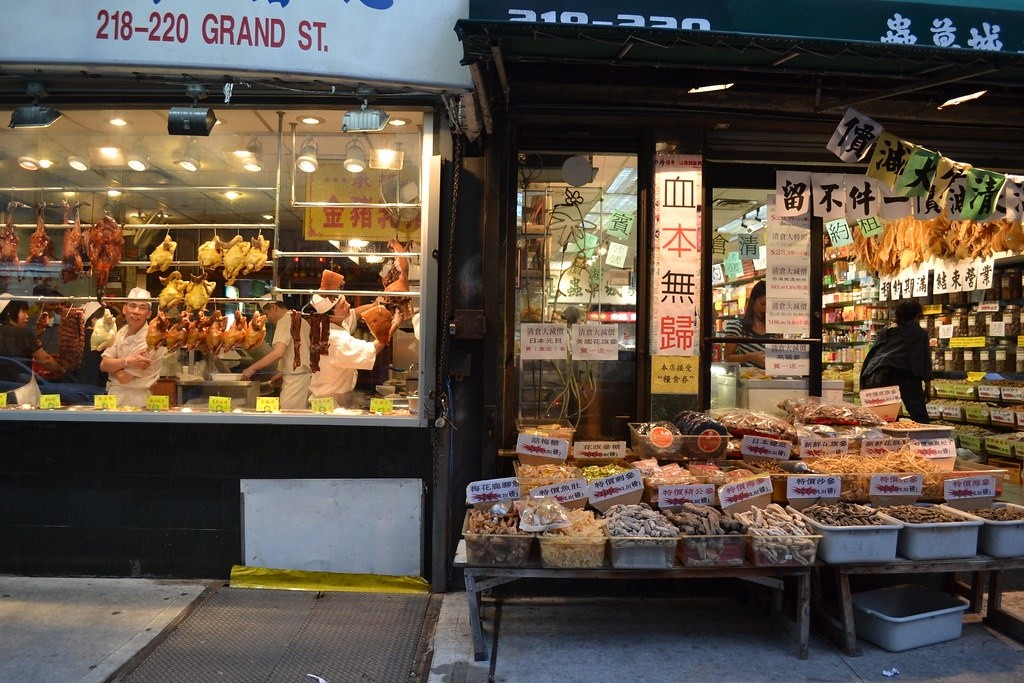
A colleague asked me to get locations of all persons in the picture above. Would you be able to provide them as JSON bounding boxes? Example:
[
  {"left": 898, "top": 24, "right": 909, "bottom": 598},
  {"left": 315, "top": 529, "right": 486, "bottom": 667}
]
[
  {"left": 308, "top": 288, "right": 403, "bottom": 404},
  {"left": 0, "top": 293, "right": 67, "bottom": 405},
  {"left": 29, "top": 284, "right": 109, "bottom": 406},
  {"left": 560, "top": 305, "right": 580, "bottom": 365},
  {"left": 724, "top": 281, "right": 779, "bottom": 369},
  {"left": 240, "top": 293, "right": 311, "bottom": 409},
  {"left": 100, "top": 287, "right": 163, "bottom": 407},
  {"left": 860, "top": 300, "right": 933, "bottom": 425}
]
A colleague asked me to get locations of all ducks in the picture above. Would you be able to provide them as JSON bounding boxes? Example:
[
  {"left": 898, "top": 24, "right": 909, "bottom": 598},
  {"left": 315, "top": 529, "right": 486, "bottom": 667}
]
[
  {"left": 83, "top": 214, "right": 125, "bottom": 307},
  {"left": 0, "top": 201, "right": 31, "bottom": 270},
  {"left": 60, "top": 199, "right": 92, "bottom": 283},
  {"left": 26, "top": 202, "right": 61, "bottom": 268}
]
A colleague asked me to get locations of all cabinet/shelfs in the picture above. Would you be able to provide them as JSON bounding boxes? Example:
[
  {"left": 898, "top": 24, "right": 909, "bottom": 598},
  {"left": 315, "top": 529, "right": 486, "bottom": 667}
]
[
  {"left": 716, "top": 255, "right": 892, "bottom": 396},
  {"left": 518, "top": 183, "right": 569, "bottom": 416}
]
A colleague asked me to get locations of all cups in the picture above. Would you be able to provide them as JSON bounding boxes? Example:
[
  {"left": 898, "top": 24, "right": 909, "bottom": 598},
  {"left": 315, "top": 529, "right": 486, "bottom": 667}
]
[
  {"left": 409, "top": 369, "right": 418, "bottom": 379},
  {"left": 394, "top": 384, "right": 406, "bottom": 394},
  {"left": 397, "top": 372, "right": 408, "bottom": 379},
  {"left": 407, "top": 397, "right": 418, "bottom": 412}
]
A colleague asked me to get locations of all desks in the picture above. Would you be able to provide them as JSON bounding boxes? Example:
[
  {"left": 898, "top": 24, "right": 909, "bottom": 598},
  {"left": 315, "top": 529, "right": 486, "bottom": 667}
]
[
  {"left": 819, "top": 555, "right": 1023, "bottom": 657},
  {"left": 450, "top": 536, "right": 815, "bottom": 662}
]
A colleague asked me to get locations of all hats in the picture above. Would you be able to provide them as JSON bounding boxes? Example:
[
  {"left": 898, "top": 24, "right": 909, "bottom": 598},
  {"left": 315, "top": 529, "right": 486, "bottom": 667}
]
[
  {"left": 310, "top": 288, "right": 341, "bottom": 314},
  {"left": 259, "top": 292, "right": 284, "bottom": 308},
  {"left": 128, "top": 288, "right": 152, "bottom": 306},
  {"left": 82, "top": 300, "right": 102, "bottom": 324},
  {"left": 0, "top": 292, "right": 13, "bottom": 314}
]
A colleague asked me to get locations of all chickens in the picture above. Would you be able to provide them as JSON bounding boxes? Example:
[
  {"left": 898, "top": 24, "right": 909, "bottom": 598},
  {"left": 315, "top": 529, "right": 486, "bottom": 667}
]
[
  {"left": 166, "top": 311, "right": 267, "bottom": 357},
  {"left": 145, "top": 311, "right": 170, "bottom": 349},
  {"left": 89, "top": 308, "right": 117, "bottom": 351},
  {"left": 198, "top": 235, "right": 271, "bottom": 286},
  {"left": 158, "top": 271, "right": 216, "bottom": 312},
  {"left": 147, "top": 235, "right": 178, "bottom": 273}
]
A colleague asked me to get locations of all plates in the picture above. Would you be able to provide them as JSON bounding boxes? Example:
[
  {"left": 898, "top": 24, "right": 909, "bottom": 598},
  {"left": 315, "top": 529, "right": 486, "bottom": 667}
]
[{"left": 209, "top": 373, "right": 242, "bottom": 381}]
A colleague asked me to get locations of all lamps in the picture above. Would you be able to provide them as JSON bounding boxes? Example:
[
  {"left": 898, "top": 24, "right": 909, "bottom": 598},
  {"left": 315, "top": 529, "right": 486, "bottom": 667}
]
[
  {"left": 554, "top": 149, "right": 600, "bottom": 186},
  {"left": 682, "top": 65, "right": 742, "bottom": 97},
  {"left": 340, "top": 93, "right": 390, "bottom": 131},
  {"left": 7, "top": 70, "right": 66, "bottom": 128},
  {"left": 933, "top": 77, "right": 992, "bottom": 114},
  {"left": 167, "top": 83, "right": 218, "bottom": 137},
  {"left": 17, "top": 138, "right": 408, "bottom": 268}
]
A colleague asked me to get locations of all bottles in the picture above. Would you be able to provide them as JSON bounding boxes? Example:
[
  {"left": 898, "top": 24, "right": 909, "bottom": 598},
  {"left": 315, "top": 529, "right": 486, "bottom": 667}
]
[
  {"left": 918, "top": 269, "right": 1024, "bottom": 374},
  {"left": 867, "top": 304, "right": 888, "bottom": 319},
  {"left": 822, "top": 342, "right": 874, "bottom": 362}
]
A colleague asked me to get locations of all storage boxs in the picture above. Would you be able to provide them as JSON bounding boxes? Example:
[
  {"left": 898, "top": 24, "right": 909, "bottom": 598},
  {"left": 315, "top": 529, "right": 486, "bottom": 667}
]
[
  {"left": 850, "top": 584, "right": 971, "bottom": 653},
  {"left": 447, "top": 420, "right": 1024, "bottom": 569}
]
